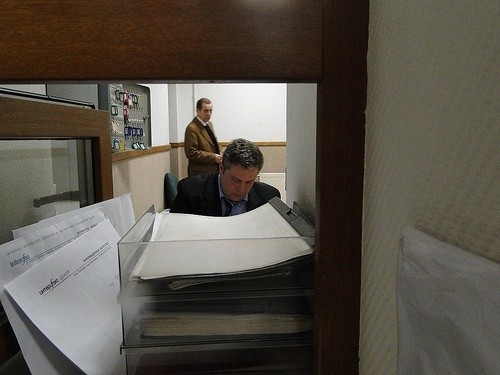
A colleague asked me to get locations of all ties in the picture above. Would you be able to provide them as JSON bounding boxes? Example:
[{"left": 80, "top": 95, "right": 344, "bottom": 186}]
[{"left": 223, "top": 197, "right": 245, "bottom": 217}]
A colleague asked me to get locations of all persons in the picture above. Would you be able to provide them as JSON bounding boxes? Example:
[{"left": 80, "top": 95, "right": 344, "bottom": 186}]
[
  {"left": 169, "top": 138, "right": 281, "bottom": 217},
  {"left": 184, "top": 99, "right": 223, "bottom": 179}
]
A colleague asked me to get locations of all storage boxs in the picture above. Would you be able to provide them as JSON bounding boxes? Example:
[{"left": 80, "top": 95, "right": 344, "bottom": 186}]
[{"left": 117, "top": 205, "right": 317, "bottom": 375}]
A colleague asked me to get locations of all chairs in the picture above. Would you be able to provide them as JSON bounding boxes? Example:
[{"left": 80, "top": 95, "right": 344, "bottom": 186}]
[{"left": 164, "top": 172, "right": 179, "bottom": 208}]
